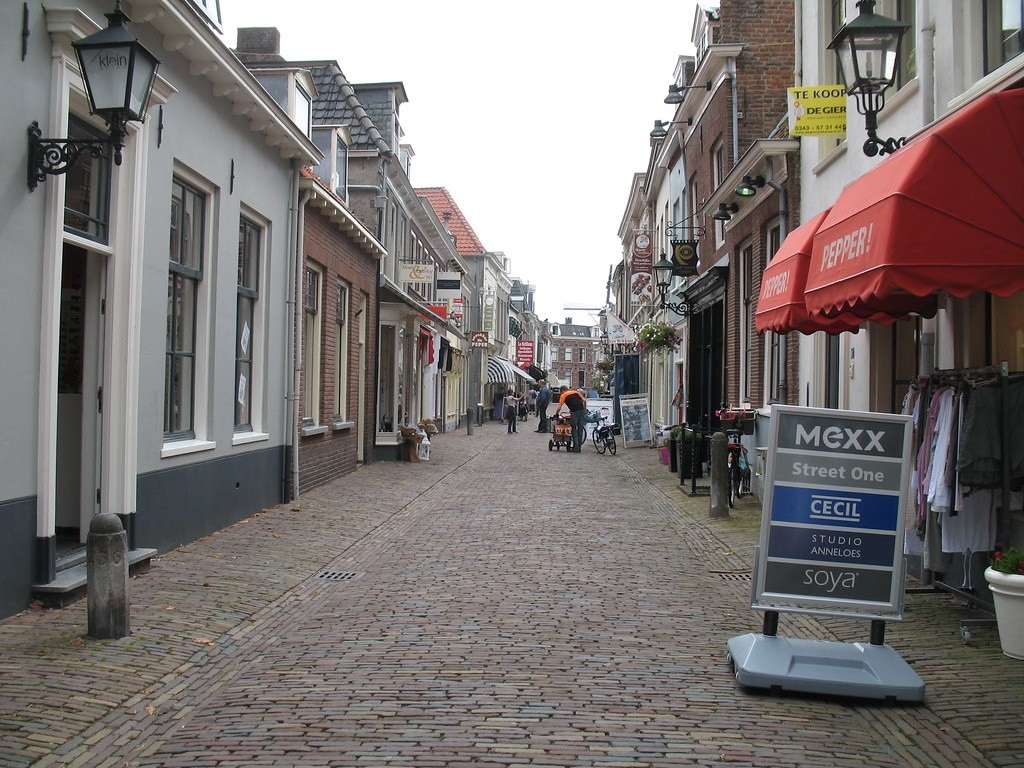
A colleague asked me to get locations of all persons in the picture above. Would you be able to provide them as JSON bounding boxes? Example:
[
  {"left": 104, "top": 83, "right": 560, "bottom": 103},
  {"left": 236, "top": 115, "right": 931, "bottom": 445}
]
[
  {"left": 518, "top": 391, "right": 527, "bottom": 422},
  {"left": 495, "top": 388, "right": 507, "bottom": 425},
  {"left": 503, "top": 389, "right": 526, "bottom": 434},
  {"left": 556, "top": 385, "right": 586, "bottom": 453},
  {"left": 534, "top": 379, "right": 550, "bottom": 433},
  {"left": 527, "top": 385, "right": 537, "bottom": 415},
  {"left": 577, "top": 386, "right": 585, "bottom": 396}
]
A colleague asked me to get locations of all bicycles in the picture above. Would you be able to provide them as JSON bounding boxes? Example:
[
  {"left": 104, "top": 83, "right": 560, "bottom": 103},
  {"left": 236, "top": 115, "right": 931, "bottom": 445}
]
[
  {"left": 584, "top": 406, "right": 618, "bottom": 455},
  {"left": 718, "top": 402, "right": 758, "bottom": 510}
]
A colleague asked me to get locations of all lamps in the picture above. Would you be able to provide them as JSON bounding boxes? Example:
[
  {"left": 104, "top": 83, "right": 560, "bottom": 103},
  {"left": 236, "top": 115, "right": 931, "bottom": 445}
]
[
  {"left": 735, "top": 175, "right": 763, "bottom": 197},
  {"left": 826, "top": 0, "right": 907, "bottom": 155},
  {"left": 664, "top": 80, "right": 712, "bottom": 105},
  {"left": 650, "top": 118, "right": 693, "bottom": 137},
  {"left": 712, "top": 202, "right": 739, "bottom": 220},
  {"left": 26, "top": 0, "right": 163, "bottom": 193},
  {"left": 650, "top": 246, "right": 695, "bottom": 315}
]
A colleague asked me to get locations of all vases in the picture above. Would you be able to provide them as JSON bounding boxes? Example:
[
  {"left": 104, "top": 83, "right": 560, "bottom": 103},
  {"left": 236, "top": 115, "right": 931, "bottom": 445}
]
[{"left": 984, "top": 566, "right": 1024, "bottom": 660}]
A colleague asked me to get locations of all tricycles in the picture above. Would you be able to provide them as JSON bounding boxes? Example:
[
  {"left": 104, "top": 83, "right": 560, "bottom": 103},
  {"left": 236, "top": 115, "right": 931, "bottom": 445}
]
[{"left": 549, "top": 413, "right": 572, "bottom": 451}]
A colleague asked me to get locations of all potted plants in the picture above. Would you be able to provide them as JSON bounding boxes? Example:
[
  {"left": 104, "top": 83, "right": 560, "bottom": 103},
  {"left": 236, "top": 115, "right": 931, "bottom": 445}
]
[
  {"left": 662, "top": 438, "right": 668, "bottom": 465},
  {"left": 658, "top": 439, "right": 666, "bottom": 461},
  {"left": 675, "top": 428, "right": 704, "bottom": 478},
  {"left": 667, "top": 425, "right": 681, "bottom": 473}
]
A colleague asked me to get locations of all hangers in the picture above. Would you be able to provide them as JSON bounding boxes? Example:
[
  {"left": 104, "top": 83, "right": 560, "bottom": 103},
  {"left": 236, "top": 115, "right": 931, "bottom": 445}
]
[{"left": 908, "top": 365, "right": 1024, "bottom": 390}]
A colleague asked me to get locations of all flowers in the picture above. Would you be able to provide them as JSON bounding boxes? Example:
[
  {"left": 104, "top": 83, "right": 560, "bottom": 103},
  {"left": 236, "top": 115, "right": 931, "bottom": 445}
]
[
  {"left": 986, "top": 547, "right": 1024, "bottom": 576},
  {"left": 602, "top": 375, "right": 611, "bottom": 383},
  {"left": 632, "top": 318, "right": 683, "bottom": 355},
  {"left": 595, "top": 357, "right": 614, "bottom": 373}
]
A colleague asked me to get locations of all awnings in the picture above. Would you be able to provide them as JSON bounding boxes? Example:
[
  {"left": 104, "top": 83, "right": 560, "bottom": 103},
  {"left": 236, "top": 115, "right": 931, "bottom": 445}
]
[
  {"left": 755, "top": 206, "right": 938, "bottom": 336},
  {"left": 804, "top": 88, "right": 1023, "bottom": 319}
]
